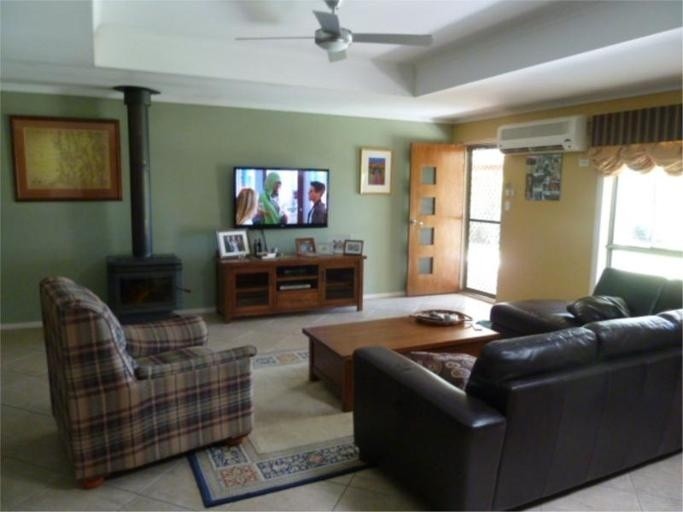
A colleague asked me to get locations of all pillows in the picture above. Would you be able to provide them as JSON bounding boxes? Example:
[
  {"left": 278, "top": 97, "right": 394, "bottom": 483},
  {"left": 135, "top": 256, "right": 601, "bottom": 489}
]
[
  {"left": 410, "top": 345, "right": 480, "bottom": 390},
  {"left": 565, "top": 293, "right": 632, "bottom": 326}
]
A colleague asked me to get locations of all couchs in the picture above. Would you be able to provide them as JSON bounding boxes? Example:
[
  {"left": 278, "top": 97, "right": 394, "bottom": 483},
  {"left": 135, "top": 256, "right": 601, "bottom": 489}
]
[
  {"left": 36, "top": 274, "right": 259, "bottom": 491},
  {"left": 488, "top": 266, "right": 683, "bottom": 334},
  {"left": 348, "top": 306, "right": 682, "bottom": 512}
]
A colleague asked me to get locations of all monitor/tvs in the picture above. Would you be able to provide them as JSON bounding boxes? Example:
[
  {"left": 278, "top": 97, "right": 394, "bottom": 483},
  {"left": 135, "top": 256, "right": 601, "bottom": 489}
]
[{"left": 233, "top": 166, "right": 328, "bottom": 228}]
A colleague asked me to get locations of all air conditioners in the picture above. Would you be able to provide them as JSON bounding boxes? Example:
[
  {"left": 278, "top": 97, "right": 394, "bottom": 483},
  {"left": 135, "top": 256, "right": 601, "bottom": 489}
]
[{"left": 496, "top": 114, "right": 590, "bottom": 157}]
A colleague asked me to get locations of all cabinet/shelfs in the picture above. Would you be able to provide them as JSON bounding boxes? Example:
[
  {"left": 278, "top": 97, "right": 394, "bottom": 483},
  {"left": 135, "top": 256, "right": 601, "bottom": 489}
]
[{"left": 216, "top": 252, "right": 366, "bottom": 326}]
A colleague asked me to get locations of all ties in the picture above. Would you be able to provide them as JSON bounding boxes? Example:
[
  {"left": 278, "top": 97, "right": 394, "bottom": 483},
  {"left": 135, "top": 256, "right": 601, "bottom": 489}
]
[{"left": 309, "top": 208, "right": 315, "bottom": 224}]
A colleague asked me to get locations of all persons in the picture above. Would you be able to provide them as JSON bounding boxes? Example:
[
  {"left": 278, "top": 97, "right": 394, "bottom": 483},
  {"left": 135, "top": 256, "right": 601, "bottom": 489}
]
[
  {"left": 236, "top": 172, "right": 327, "bottom": 225},
  {"left": 224, "top": 236, "right": 245, "bottom": 252}
]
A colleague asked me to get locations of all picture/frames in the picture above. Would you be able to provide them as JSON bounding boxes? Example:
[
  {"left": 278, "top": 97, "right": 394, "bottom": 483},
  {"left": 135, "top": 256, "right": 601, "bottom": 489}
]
[
  {"left": 326, "top": 233, "right": 352, "bottom": 254},
  {"left": 356, "top": 146, "right": 393, "bottom": 197},
  {"left": 343, "top": 239, "right": 363, "bottom": 256},
  {"left": 316, "top": 241, "right": 334, "bottom": 256},
  {"left": 294, "top": 237, "right": 316, "bottom": 255},
  {"left": 7, "top": 112, "right": 122, "bottom": 204},
  {"left": 214, "top": 228, "right": 251, "bottom": 259}
]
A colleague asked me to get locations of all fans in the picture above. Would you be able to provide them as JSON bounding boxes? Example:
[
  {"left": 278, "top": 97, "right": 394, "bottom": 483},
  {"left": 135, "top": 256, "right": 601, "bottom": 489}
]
[{"left": 232, "top": 0, "right": 437, "bottom": 63}]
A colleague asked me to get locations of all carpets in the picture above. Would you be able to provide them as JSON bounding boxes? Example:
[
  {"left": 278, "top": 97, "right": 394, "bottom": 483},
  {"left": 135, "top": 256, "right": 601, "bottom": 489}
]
[{"left": 185, "top": 332, "right": 382, "bottom": 509}]
